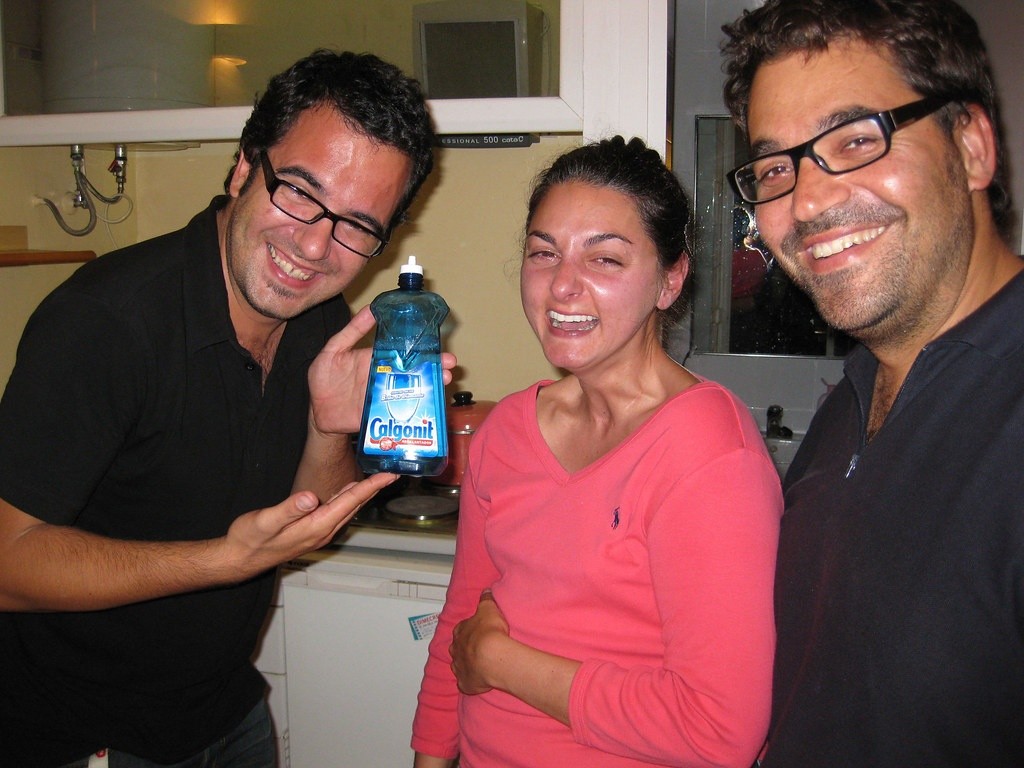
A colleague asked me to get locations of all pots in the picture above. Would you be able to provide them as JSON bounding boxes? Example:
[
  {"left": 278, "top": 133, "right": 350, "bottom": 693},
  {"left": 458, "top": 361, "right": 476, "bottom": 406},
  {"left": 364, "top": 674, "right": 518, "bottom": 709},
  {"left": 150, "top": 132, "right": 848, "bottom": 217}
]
[{"left": 432, "top": 392, "right": 498, "bottom": 487}]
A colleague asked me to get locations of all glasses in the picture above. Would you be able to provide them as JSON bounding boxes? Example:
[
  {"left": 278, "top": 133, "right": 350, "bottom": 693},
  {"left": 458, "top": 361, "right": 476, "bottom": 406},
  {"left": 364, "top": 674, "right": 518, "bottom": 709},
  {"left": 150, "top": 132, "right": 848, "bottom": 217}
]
[
  {"left": 257, "top": 146, "right": 390, "bottom": 258},
  {"left": 725, "top": 96, "right": 950, "bottom": 207}
]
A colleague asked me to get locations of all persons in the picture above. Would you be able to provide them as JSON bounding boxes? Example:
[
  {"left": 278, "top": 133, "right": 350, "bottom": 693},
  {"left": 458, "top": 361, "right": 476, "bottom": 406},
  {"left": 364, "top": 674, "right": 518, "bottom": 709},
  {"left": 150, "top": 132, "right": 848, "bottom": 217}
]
[
  {"left": 2, "top": 45, "right": 463, "bottom": 768},
  {"left": 724, "top": 0, "right": 1024, "bottom": 768},
  {"left": 409, "top": 135, "right": 785, "bottom": 768}
]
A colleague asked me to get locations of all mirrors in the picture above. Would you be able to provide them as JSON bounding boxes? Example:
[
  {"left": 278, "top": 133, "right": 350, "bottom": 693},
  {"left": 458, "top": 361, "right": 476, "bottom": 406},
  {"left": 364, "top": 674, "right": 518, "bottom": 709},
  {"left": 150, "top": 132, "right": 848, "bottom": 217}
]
[{"left": 689, "top": 113, "right": 864, "bottom": 360}]
